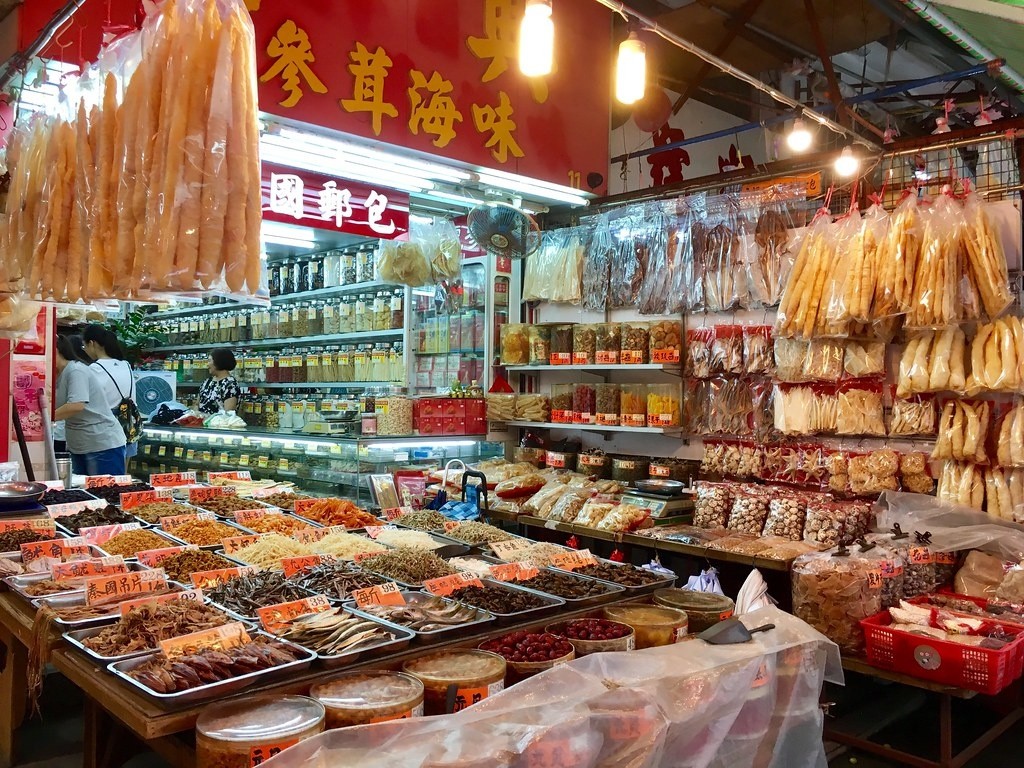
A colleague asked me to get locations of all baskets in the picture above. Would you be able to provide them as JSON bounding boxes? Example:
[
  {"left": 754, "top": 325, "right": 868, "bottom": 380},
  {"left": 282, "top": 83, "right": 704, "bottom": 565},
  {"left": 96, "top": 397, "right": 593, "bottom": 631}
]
[{"left": 855, "top": 591, "right": 1023, "bottom": 696}]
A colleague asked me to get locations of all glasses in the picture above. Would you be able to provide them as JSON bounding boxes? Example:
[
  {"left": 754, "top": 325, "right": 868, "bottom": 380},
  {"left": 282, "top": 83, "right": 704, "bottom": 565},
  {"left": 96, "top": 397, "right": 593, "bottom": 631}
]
[{"left": 81, "top": 340, "right": 95, "bottom": 351}]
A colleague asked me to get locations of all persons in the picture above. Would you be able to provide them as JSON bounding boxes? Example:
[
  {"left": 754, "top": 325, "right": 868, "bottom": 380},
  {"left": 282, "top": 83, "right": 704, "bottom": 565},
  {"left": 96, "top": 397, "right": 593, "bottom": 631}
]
[
  {"left": 196, "top": 348, "right": 240, "bottom": 415},
  {"left": 56, "top": 322, "right": 138, "bottom": 476}
]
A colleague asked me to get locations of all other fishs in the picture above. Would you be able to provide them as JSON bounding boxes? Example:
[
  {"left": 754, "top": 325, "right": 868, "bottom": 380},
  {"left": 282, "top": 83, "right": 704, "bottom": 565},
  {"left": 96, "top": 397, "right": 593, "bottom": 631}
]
[
  {"left": 274, "top": 611, "right": 396, "bottom": 657},
  {"left": 358, "top": 595, "right": 491, "bottom": 635}
]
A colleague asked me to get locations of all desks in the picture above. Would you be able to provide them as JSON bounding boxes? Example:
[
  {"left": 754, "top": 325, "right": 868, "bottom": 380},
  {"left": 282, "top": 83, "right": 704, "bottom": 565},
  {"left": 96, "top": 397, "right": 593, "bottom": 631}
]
[
  {"left": 621, "top": 533, "right": 795, "bottom": 573},
  {"left": 0, "top": 580, "right": 678, "bottom": 768},
  {"left": 819, "top": 650, "right": 1024, "bottom": 768},
  {"left": 480, "top": 508, "right": 517, "bottom": 522},
  {"left": 517, "top": 513, "right": 623, "bottom": 546},
  {"left": 0, "top": 586, "right": 66, "bottom": 768}
]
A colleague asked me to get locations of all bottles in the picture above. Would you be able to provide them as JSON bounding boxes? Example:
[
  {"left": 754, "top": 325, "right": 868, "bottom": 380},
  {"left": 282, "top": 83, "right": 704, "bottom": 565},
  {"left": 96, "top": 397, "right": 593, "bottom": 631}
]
[
  {"left": 477, "top": 630, "right": 575, "bottom": 689},
  {"left": 127, "top": 241, "right": 415, "bottom": 436},
  {"left": 602, "top": 601, "right": 689, "bottom": 651},
  {"left": 501, "top": 319, "right": 682, "bottom": 426},
  {"left": 55, "top": 451, "right": 73, "bottom": 489},
  {"left": 514, "top": 448, "right": 692, "bottom": 484},
  {"left": 545, "top": 617, "right": 636, "bottom": 665},
  {"left": 403, "top": 646, "right": 508, "bottom": 715},
  {"left": 195, "top": 691, "right": 328, "bottom": 768},
  {"left": 309, "top": 669, "right": 424, "bottom": 732}
]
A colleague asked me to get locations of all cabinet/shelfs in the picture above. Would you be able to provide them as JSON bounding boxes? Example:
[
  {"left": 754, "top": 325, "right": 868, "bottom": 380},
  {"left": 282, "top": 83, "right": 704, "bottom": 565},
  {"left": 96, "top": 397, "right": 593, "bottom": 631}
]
[
  {"left": 504, "top": 364, "right": 691, "bottom": 446},
  {"left": 143, "top": 256, "right": 490, "bottom": 393},
  {"left": 127, "top": 424, "right": 506, "bottom": 510}
]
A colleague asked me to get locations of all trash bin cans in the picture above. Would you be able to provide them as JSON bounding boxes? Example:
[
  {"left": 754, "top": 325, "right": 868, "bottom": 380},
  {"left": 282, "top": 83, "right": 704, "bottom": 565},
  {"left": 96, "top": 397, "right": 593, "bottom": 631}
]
[{"left": 55, "top": 452, "right": 73, "bottom": 489}]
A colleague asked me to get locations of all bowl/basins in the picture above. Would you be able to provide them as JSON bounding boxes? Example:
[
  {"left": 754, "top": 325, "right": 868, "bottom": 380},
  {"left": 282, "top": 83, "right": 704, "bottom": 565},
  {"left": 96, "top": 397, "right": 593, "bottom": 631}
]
[
  {"left": 635, "top": 478, "right": 686, "bottom": 494},
  {"left": 0, "top": 482, "right": 48, "bottom": 505}
]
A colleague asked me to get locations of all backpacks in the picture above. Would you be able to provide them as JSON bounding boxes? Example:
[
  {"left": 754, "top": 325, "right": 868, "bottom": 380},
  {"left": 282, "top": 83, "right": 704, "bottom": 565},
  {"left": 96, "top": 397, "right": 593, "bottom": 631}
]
[{"left": 95, "top": 360, "right": 145, "bottom": 445}]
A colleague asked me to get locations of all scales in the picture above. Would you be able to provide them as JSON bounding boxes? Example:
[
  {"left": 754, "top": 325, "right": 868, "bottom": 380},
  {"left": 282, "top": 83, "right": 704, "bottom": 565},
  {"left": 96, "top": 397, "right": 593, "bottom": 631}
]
[{"left": 620, "top": 479, "right": 698, "bottom": 528}]
